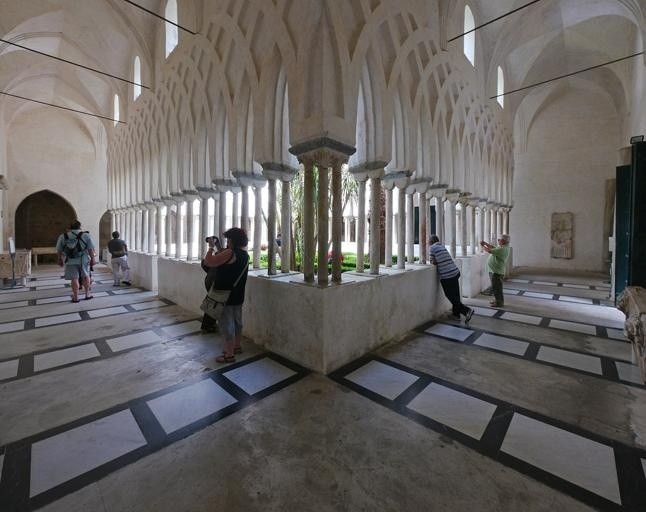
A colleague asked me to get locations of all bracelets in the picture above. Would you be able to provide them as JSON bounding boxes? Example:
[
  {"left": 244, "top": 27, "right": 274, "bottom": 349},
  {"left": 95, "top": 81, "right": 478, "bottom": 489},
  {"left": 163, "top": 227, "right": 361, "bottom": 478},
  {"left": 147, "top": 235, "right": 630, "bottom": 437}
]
[{"left": 208, "top": 245, "right": 218, "bottom": 253}]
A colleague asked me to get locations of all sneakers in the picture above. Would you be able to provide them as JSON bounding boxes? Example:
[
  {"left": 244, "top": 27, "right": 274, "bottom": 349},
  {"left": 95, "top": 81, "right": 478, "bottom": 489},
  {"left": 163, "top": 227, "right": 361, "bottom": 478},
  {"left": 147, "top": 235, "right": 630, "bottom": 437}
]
[
  {"left": 70, "top": 280, "right": 132, "bottom": 304},
  {"left": 490, "top": 301, "right": 504, "bottom": 308},
  {"left": 202, "top": 322, "right": 243, "bottom": 363},
  {"left": 448, "top": 308, "right": 475, "bottom": 323}
]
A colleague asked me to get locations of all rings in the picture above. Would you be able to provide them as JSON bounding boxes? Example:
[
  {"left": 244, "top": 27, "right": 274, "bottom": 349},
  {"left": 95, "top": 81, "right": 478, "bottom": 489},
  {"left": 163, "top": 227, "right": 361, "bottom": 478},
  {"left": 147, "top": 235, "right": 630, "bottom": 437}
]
[{"left": 211, "top": 239, "right": 213, "bottom": 242}]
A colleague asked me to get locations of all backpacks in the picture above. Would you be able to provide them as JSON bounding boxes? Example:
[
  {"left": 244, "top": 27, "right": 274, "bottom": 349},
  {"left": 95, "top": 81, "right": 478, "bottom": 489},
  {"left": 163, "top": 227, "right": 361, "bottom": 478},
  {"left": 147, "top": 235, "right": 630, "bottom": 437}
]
[{"left": 63, "top": 232, "right": 84, "bottom": 259}]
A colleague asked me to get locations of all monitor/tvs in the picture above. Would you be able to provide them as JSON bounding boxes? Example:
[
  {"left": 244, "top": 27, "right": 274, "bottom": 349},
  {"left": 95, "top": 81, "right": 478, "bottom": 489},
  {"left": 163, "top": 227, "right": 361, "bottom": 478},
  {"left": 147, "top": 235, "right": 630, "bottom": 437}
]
[{"left": 206, "top": 236, "right": 218, "bottom": 244}]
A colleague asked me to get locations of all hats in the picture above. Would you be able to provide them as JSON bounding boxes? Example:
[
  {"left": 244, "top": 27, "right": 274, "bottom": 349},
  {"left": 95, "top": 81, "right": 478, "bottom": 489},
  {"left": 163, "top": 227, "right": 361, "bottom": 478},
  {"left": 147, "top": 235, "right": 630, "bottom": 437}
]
[{"left": 498, "top": 234, "right": 512, "bottom": 243}]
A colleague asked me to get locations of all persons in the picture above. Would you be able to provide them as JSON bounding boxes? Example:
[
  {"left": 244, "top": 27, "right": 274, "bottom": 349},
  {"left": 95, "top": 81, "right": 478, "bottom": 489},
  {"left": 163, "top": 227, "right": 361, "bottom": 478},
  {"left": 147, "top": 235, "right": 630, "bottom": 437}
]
[
  {"left": 55, "top": 220, "right": 96, "bottom": 302},
  {"left": 204, "top": 227, "right": 251, "bottom": 363},
  {"left": 478, "top": 233, "right": 511, "bottom": 308},
  {"left": 276, "top": 233, "right": 282, "bottom": 263},
  {"left": 78, "top": 229, "right": 97, "bottom": 291},
  {"left": 201, "top": 235, "right": 226, "bottom": 333},
  {"left": 107, "top": 230, "right": 131, "bottom": 287},
  {"left": 428, "top": 234, "right": 475, "bottom": 324}
]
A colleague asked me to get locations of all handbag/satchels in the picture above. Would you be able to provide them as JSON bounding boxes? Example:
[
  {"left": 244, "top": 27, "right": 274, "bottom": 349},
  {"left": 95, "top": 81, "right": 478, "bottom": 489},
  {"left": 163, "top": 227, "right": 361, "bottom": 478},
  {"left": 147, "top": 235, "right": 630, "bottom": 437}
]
[
  {"left": 200, "top": 286, "right": 232, "bottom": 320},
  {"left": 112, "top": 251, "right": 124, "bottom": 256}
]
